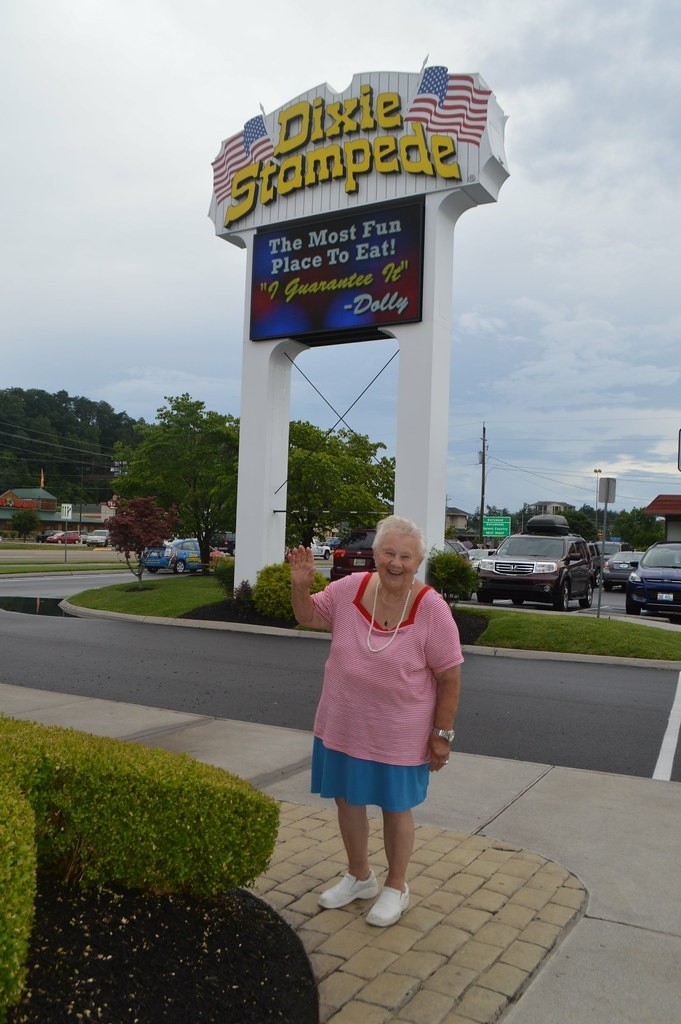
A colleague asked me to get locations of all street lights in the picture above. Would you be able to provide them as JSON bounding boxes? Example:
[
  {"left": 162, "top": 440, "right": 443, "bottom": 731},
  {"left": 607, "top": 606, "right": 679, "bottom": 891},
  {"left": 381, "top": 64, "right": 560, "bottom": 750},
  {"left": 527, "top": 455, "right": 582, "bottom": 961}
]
[{"left": 594, "top": 468, "right": 602, "bottom": 530}]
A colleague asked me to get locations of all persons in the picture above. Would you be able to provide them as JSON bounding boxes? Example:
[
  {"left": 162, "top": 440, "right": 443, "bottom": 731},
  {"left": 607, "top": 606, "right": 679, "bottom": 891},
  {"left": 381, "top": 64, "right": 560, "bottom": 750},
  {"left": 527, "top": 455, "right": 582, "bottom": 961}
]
[{"left": 289, "top": 516, "right": 465, "bottom": 928}]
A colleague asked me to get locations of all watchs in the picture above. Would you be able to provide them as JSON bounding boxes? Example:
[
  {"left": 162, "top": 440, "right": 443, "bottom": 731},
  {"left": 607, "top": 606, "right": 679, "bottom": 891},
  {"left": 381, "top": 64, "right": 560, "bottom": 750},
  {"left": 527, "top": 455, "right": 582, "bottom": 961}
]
[{"left": 430, "top": 727, "right": 456, "bottom": 742}]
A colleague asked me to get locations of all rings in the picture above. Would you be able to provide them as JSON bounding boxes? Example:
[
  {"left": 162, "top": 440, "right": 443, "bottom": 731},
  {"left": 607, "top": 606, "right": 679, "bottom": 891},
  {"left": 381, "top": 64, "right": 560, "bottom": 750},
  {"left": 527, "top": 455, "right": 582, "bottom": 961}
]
[{"left": 443, "top": 760, "right": 448, "bottom": 765}]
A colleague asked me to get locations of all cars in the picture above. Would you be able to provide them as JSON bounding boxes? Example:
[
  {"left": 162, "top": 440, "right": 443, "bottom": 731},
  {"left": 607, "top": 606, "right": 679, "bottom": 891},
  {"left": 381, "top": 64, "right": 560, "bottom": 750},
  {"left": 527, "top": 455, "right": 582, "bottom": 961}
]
[
  {"left": 330, "top": 527, "right": 378, "bottom": 583},
  {"left": 448, "top": 540, "right": 470, "bottom": 562},
  {"left": 602, "top": 552, "right": 647, "bottom": 591},
  {"left": 45, "top": 531, "right": 81, "bottom": 544},
  {"left": 594, "top": 542, "right": 633, "bottom": 565},
  {"left": 287, "top": 538, "right": 331, "bottom": 560},
  {"left": 36, "top": 530, "right": 62, "bottom": 544},
  {"left": 587, "top": 543, "right": 604, "bottom": 585},
  {"left": 326, "top": 537, "right": 344, "bottom": 549},
  {"left": 78, "top": 532, "right": 89, "bottom": 543},
  {"left": 464, "top": 548, "right": 506, "bottom": 571},
  {"left": 444, "top": 539, "right": 460, "bottom": 556},
  {"left": 85, "top": 530, "right": 116, "bottom": 547},
  {"left": 142, "top": 538, "right": 222, "bottom": 574},
  {"left": 626, "top": 540, "right": 681, "bottom": 615}
]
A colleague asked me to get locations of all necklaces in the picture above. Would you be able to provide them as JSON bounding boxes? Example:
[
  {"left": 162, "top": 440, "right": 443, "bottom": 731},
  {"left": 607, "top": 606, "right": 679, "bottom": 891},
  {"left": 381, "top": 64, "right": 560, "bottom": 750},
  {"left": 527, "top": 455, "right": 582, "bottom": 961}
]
[{"left": 366, "top": 573, "right": 416, "bottom": 653}]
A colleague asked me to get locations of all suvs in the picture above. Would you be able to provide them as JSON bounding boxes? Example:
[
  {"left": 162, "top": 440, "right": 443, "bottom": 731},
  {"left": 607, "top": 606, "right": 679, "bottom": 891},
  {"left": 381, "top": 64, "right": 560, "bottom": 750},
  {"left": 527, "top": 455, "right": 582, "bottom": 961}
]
[{"left": 475, "top": 515, "right": 595, "bottom": 611}]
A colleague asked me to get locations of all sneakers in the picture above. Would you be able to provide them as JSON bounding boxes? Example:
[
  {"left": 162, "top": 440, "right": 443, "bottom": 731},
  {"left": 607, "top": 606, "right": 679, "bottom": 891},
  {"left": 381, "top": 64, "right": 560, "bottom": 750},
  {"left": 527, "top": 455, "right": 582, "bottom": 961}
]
[
  {"left": 318, "top": 870, "right": 378, "bottom": 909},
  {"left": 366, "top": 881, "right": 409, "bottom": 926}
]
